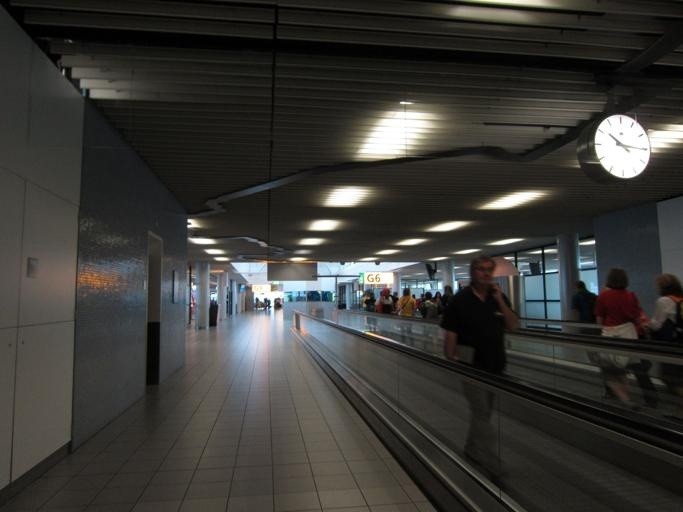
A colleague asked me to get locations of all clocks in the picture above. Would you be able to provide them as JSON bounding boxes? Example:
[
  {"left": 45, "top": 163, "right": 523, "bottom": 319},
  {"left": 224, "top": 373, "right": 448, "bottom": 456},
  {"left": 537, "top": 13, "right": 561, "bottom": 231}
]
[{"left": 576, "top": 112, "right": 651, "bottom": 186}]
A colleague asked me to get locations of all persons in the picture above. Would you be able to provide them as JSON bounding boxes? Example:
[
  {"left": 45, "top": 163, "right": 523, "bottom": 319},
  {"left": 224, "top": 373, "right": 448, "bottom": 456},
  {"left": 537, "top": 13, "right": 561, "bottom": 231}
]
[
  {"left": 647, "top": 273, "right": 682, "bottom": 426},
  {"left": 568, "top": 280, "right": 599, "bottom": 365},
  {"left": 630, "top": 306, "right": 660, "bottom": 411},
  {"left": 359, "top": 283, "right": 454, "bottom": 355},
  {"left": 593, "top": 269, "right": 642, "bottom": 418},
  {"left": 439, "top": 256, "right": 520, "bottom": 478}
]
